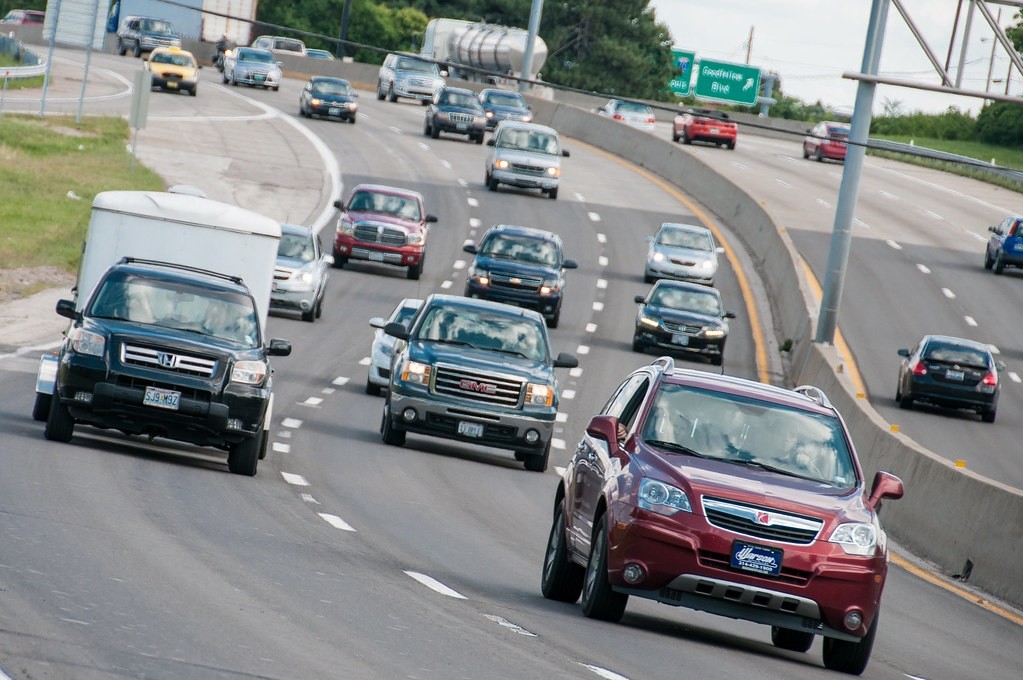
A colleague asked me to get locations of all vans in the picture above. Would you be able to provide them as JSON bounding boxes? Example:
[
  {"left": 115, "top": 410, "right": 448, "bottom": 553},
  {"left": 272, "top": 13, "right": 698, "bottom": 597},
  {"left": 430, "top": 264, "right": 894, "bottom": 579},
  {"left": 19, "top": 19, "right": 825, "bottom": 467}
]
[
  {"left": 248, "top": 35, "right": 310, "bottom": 58},
  {"left": 485, "top": 121, "right": 570, "bottom": 200}
]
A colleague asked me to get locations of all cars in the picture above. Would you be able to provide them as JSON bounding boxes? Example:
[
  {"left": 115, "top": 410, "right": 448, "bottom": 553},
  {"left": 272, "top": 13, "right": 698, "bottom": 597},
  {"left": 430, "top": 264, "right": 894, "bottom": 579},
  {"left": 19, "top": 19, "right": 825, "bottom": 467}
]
[
  {"left": 307, "top": 48, "right": 341, "bottom": 63},
  {"left": 474, "top": 87, "right": 534, "bottom": 133},
  {"left": 985, "top": 216, "right": 1023, "bottom": 273},
  {"left": 634, "top": 280, "right": 737, "bottom": 367},
  {"left": 299, "top": 76, "right": 362, "bottom": 126},
  {"left": 138, "top": 45, "right": 203, "bottom": 96},
  {"left": 801, "top": 120, "right": 854, "bottom": 160},
  {"left": 645, "top": 222, "right": 725, "bottom": 289},
  {"left": 596, "top": 98, "right": 656, "bottom": 133},
  {"left": 672, "top": 107, "right": 737, "bottom": 149},
  {"left": 895, "top": 335, "right": 1006, "bottom": 423},
  {"left": 223, "top": 46, "right": 285, "bottom": 93},
  {"left": 365, "top": 298, "right": 426, "bottom": 396},
  {"left": 266, "top": 222, "right": 335, "bottom": 323}
]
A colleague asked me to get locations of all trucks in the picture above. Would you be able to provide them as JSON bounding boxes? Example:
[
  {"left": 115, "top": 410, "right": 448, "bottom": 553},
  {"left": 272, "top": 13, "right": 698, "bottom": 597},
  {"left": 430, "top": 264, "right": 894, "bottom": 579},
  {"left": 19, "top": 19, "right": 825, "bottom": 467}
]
[{"left": 31, "top": 187, "right": 276, "bottom": 457}]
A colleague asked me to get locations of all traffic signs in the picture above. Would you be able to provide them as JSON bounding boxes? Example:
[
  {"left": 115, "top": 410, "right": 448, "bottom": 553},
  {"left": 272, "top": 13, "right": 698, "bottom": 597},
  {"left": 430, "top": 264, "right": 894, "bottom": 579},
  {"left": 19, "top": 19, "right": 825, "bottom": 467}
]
[{"left": 695, "top": 59, "right": 762, "bottom": 106}]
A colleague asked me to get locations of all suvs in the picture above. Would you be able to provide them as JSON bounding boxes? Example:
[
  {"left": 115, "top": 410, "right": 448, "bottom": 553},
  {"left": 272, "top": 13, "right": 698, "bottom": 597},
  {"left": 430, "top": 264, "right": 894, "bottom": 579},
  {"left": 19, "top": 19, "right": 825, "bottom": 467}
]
[
  {"left": 332, "top": 183, "right": 442, "bottom": 280},
  {"left": 382, "top": 293, "right": 582, "bottom": 473},
  {"left": 422, "top": 84, "right": 491, "bottom": 147},
  {"left": 539, "top": 356, "right": 906, "bottom": 675},
  {"left": 377, "top": 51, "right": 447, "bottom": 104},
  {"left": 45, "top": 254, "right": 293, "bottom": 476},
  {"left": 0, "top": 8, "right": 48, "bottom": 29},
  {"left": 461, "top": 221, "right": 579, "bottom": 331},
  {"left": 113, "top": 14, "right": 186, "bottom": 58}
]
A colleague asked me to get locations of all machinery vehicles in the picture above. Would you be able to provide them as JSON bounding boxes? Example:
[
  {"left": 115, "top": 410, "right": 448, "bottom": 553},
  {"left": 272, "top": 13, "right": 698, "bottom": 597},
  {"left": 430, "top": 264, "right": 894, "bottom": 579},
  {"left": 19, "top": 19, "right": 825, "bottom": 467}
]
[{"left": 421, "top": 15, "right": 554, "bottom": 103}]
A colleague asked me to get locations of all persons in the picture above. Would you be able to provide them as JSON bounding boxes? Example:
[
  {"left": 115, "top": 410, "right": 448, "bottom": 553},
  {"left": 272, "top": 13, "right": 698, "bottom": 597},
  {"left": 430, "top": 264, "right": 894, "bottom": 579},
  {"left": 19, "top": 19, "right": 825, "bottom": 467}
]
[
  {"left": 216, "top": 33, "right": 233, "bottom": 54},
  {"left": 179, "top": 301, "right": 237, "bottom": 341},
  {"left": 744, "top": 419, "right": 814, "bottom": 474}
]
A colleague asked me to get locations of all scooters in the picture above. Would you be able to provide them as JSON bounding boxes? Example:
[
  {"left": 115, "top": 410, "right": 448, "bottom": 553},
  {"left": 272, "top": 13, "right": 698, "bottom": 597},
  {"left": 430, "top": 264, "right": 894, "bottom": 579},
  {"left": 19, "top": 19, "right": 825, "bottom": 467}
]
[{"left": 213, "top": 47, "right": 229, "bottom": 74}]
